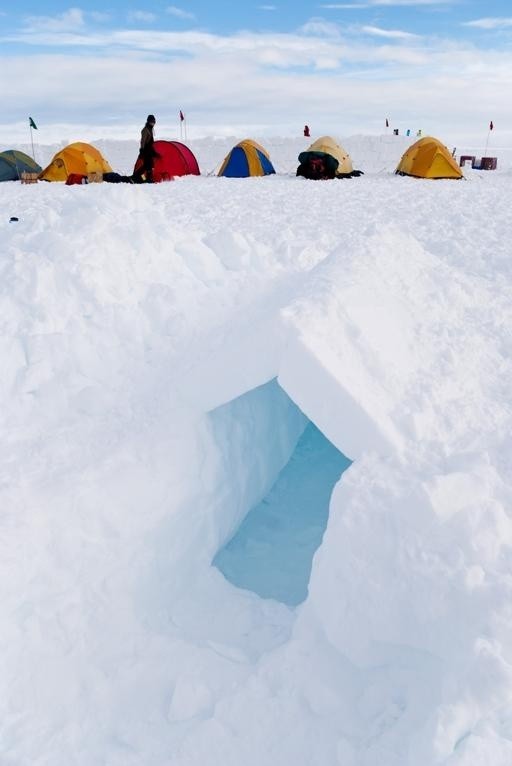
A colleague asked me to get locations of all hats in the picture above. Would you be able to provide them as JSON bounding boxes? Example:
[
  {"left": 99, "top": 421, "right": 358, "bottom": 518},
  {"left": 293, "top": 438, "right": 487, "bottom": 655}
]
[{"left": 147, "top": 115, "right": 156, "bottom": 123}]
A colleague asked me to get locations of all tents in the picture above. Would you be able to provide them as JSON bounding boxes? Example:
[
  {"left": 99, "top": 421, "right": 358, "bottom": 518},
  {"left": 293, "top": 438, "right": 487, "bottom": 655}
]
[
  {"left": 296, "top": 136, "right": 354, "bottom": 177},
  {"left": 134, "top": 140, "right": 200, "bottom": 182},
  {"left": 217, "top": 138, "right": 276, "bottom": 178},
  {"left": 0, "top": 150, "right": 42, "bottom": 182},
  {"left": 37, "top": 142, "right": 114, "bottom": 184},
  {"left": 395, "top": 135, "right": 466, "bottom": 180}
]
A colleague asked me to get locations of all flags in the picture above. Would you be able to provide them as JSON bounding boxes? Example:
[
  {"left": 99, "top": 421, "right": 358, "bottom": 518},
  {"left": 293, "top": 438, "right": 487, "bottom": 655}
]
[
  {"left": 180, "top": 110, "right": 185, "bottom": 122},
  {"left": 29, "top": 117, "right": 38, "bottom": 130},
  {"left": 490, "top": 121, "right": 493, "bottom": 130}
]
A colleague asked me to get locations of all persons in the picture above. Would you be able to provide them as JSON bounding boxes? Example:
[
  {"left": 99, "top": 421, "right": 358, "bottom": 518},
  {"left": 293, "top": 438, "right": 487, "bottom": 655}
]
[
  {"left": 393, "top": 129, "right": 422, "bottom": 136},
  {"left": 130, "top": 115, "right": 163, "bottom": 184},
  {"left": 303, "top": 126, "right": 310, "bottom": 137}
]
